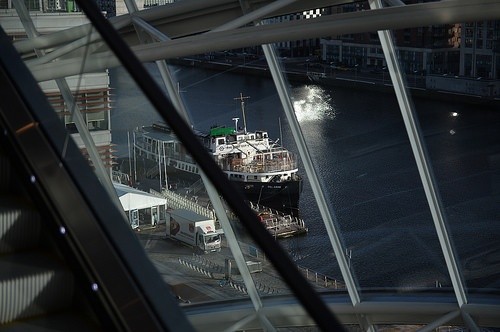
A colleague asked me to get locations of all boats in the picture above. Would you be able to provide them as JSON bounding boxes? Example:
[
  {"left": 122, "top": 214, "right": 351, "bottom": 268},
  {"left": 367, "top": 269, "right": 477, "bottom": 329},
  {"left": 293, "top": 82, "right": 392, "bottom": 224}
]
[{"left": 131, "top": 92, "right": 305, "bottom": 206}]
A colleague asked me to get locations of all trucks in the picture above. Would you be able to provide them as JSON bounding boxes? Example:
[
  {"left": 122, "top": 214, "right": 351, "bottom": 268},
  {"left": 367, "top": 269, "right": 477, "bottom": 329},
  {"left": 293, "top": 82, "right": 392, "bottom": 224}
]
[{"left": 169, "top": 208, "right": 222, "bottom": 255}]
